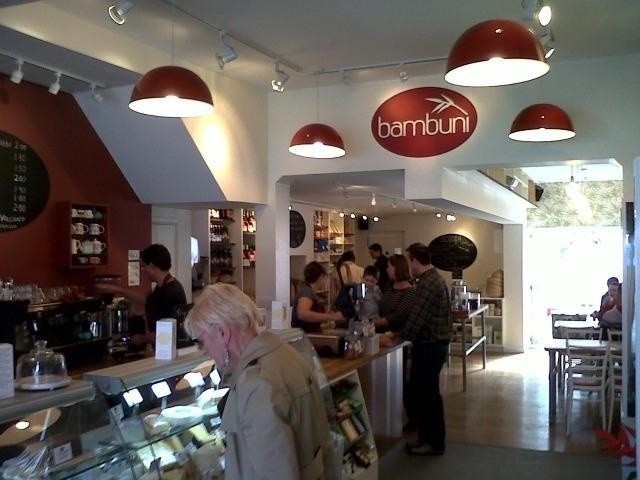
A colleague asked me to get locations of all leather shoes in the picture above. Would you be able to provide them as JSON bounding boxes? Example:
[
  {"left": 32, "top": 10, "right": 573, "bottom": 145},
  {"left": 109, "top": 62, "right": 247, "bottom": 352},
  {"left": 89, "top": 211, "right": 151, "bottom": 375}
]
[{"left": 406, "top": 441, "right": 444, "bottom": 455}]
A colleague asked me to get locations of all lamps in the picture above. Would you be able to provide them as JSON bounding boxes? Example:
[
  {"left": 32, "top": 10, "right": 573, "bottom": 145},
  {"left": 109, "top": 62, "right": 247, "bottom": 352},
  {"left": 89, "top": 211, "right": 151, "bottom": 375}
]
[
  {"left": 540, "top": 43, "right": 556, "bottom": 59},
  {"left": 49, "top": 77, "right": 61, "bottom": 95},
  {"left": 270, "top": 70, "right": 288, "bottom": 94},
  {"left": 445, "top": 1, "right": 550, "bottom": 87},
  {"left": 397, "top": 65, "right": 409, "bottom": 82},
  {"left": 215, "top": 49, "right": 237, "bottom": 69},
  {"left": 520, "top": 4, "right": 552, "bottom": 28},
  {"left": 289, "top": 85, "right": 347, "bottom": 159},
  {"left": 12, "top": 64, "right": 24, "bottom": 84},
  {"left": 89, "top": 86, "right": 105, "bottom": 103},
  {"left": 509, "top": 79, "right": 575, "bottom": 143},
  {"left": 371, "top": 191, "right": 417, "bottom": 222},
  {"left": 127, "top": 28, "right": 215, "bottom": 119},
  {"left": 105, "top": 1, "right": 136, "bottom": 26}
]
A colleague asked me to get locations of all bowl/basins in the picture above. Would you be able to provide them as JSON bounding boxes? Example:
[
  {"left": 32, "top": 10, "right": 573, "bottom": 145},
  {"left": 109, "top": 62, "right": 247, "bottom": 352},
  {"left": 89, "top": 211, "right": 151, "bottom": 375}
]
[{"left": 159, "top": 404, "right": 203, "bottom": 428}]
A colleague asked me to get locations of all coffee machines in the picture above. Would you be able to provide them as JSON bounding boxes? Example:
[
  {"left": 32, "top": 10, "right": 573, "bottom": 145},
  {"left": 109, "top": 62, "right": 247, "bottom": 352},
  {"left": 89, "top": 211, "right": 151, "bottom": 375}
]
[{"left": 94, "top": 274, "right": 121, "bottom": 339}]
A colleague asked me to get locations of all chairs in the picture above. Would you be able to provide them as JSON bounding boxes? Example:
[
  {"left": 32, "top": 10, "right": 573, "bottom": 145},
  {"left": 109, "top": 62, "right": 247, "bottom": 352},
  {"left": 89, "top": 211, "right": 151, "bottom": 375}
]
[{"left": 553, "top": 313, "right": 622, "bottom": 436}]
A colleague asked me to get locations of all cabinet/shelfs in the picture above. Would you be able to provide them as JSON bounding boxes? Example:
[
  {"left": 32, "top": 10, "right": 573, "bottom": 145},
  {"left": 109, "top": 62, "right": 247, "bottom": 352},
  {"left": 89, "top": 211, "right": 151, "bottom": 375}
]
[
  {"left": 197, "top": 206, "right": 255, "bottom": 305},
  {"left": 55, "top": 200, "right": 110, "bottom": 269},
  {"left": 451, "top": 297, "right": 505, "bottom": 355},
  {"left": 0, "top": 329, "right": 379, "bottom": 480}
]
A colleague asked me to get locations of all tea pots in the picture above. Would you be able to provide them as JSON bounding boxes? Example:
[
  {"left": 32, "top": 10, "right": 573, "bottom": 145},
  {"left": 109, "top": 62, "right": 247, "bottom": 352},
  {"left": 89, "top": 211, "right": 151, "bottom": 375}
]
[{"left": 72, "top": 208, "right": 107, "bottom": 267}]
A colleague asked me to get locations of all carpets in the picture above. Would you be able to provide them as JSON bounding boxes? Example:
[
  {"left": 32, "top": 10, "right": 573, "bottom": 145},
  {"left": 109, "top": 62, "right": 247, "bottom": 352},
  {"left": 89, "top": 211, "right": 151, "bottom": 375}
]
[{"left": 378, "top": 437, "right": 622, "bottom": 480}]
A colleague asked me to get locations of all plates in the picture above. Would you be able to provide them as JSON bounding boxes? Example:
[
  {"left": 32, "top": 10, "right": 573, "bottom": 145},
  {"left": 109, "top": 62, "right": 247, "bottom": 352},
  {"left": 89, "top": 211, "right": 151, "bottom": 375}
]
[{"left": 189, "top": 399, "right": 221, "bottom": 416}]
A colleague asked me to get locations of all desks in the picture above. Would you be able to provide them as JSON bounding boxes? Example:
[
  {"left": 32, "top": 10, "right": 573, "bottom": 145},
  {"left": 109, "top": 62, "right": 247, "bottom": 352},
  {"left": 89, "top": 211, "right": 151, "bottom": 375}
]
[
  {"left": 544, "top": 339, "right": 622, "bottom": 425},
  {"left": 446, "top": 304, "right": 489, "bottom": 392}
]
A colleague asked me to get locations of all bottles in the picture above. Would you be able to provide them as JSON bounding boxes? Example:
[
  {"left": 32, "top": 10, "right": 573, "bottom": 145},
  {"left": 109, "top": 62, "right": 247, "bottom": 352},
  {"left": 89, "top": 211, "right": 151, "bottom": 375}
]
[
  {"left": 341, "top": 310, "right": 380, "bottom": 358},
  {"left": 331, "top": 386, "right": 377, "bottom": 470},
  {"left": 314, "top": 211, "right": 329, "bottom": 251},
  {"left": 212, "top": 249, "right": 233, "bottom": 269},
  {"left": 242, "top": 208, "right": 256, "bottom": 267},
  {"left": 210, "top": 224, "right": 228, "bottom": 243}
]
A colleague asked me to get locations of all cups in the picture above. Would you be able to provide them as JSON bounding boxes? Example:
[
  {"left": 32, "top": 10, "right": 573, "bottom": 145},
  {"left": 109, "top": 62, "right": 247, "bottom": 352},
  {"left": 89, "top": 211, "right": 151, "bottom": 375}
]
[
  {"left": 0, "top": 279, "right": 92, "bottom": 304},
  {"left": 449, "top": 292, "right": 482, "bottom": 311}
]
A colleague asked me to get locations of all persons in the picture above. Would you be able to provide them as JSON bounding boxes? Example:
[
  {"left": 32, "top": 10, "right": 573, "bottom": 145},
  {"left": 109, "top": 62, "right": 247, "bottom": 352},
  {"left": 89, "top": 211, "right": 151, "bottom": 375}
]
[
  {"left": 183, "top": 280, "right": 338, "bottom": 480},
  {"left": 93, "top": 243, "right": 189, "bottom": 350},
  {"left": 290, "top": 241, "right": 454, "bottom": 457},
  {"left": 590, "top": 276, "right": 622, "bottom": 341}
]
